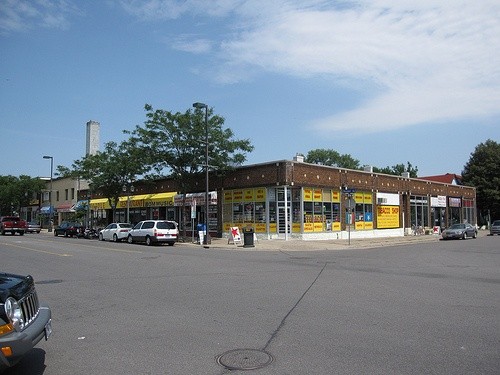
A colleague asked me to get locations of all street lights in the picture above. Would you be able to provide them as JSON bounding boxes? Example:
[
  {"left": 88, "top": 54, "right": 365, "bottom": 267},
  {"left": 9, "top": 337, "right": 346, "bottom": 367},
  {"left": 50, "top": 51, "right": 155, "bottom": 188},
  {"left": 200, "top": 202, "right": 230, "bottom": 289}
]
[
  {"left": 43, "top": 156, "right": 53, "bottom": 232},
  {"left": 192, "top": 102, "right": 208, "bottom": 245}
]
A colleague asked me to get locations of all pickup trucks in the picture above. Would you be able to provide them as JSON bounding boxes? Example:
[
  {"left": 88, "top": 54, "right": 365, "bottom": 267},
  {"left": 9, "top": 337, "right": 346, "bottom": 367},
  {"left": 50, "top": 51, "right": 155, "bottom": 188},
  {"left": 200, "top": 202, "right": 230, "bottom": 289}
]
[{"left": 0, "top": 216, "right": 26, "bottom": 236}]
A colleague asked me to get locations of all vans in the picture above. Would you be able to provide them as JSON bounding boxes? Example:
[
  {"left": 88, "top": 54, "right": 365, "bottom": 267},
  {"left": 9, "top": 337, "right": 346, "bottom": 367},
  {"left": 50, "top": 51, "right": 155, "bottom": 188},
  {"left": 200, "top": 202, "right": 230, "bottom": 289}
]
[{"left": 128, "top": 220, "right": 179, "bottom": 246}]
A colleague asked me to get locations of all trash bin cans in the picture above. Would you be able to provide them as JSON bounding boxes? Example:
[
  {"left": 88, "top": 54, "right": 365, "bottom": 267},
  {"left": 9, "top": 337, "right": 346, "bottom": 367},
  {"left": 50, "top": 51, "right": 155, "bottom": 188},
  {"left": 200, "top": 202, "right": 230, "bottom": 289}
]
[{"left": 242, "top": 227, "right": 255, "bottom": 248}]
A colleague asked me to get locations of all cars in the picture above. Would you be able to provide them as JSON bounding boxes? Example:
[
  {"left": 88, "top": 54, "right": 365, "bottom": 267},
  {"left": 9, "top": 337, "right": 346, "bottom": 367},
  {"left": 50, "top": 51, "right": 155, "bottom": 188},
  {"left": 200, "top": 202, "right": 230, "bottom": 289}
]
[
  {"left": 25, "top": 222, "right": 41, "bottom": 234},
  {"left": 54, "top": 222, "right": 85, "bottom": 237},
  {"left": 442, "top": 223, "right": 478, "bottom": 240},
  {"left": 0, "top": 273, "right": 51, "bottom": 370},
  {"left": 490, "top": 220, "right": 500, "bottom": 236},
  {"left": 99, "top": 223, "right": 134, "bottom": 242}
]
